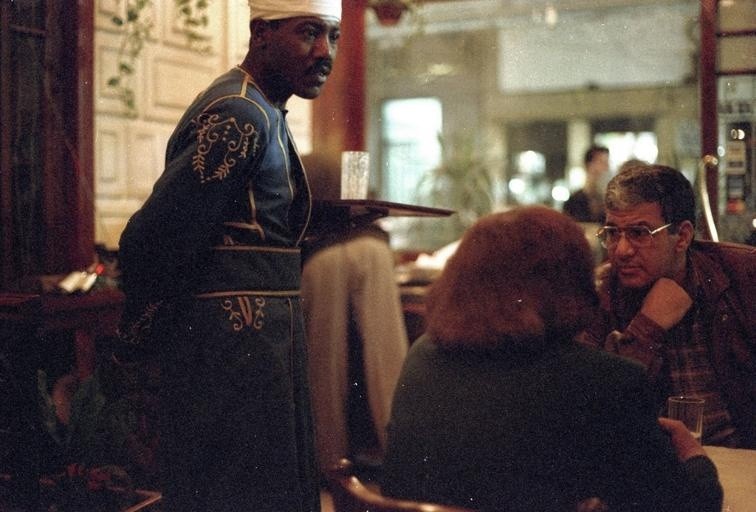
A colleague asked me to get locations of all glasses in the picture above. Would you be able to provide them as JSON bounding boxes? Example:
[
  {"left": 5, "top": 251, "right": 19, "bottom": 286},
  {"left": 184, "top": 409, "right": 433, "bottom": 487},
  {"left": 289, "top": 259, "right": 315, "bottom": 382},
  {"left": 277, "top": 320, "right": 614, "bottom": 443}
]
[{"left": 597, "top": 222, "right": 674, "bottom": 250}]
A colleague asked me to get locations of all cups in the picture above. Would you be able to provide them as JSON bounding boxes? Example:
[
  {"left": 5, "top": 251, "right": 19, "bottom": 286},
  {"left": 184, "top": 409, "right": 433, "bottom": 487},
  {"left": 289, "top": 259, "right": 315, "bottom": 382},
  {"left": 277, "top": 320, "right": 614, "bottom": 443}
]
[
  {"left": 340, "top": 150, "right": 370, "bottom": 200},
  {"left": 667, "top": 396, "right": 704, "bottom": 447}
]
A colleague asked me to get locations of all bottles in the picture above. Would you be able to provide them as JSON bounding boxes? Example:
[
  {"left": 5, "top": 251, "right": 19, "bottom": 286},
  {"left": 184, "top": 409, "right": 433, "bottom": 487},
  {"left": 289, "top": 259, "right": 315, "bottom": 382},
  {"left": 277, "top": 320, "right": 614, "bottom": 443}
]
[{"left": 52, "top": 263, "right": 106, "bottom": 298}]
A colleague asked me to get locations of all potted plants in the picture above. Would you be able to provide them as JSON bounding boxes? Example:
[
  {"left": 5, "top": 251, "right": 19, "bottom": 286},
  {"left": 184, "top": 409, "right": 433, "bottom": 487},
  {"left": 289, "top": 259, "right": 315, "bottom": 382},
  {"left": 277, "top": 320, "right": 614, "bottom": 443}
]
[{"left": 0, "top": 366, "right": 163, "bottom": 512}]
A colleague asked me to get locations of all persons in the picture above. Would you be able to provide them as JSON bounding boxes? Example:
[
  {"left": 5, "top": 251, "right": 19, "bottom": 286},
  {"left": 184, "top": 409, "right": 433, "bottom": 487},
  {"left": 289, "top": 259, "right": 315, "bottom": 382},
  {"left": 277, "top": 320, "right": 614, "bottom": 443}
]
[
  {"left": 563, "top": 149, "right": 612, "bottom": 222},
  {"left": 380, "top": 206, "right": 724, "bottom": 512},
  {"left": 595, "top": 155, "right": 755, "bottom": 450},
  {"left": 117, "top": 1, "right": 347, "bottom": 511}
]
